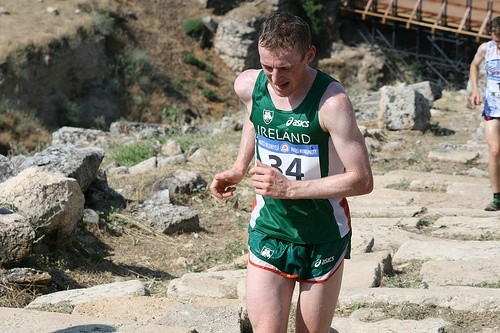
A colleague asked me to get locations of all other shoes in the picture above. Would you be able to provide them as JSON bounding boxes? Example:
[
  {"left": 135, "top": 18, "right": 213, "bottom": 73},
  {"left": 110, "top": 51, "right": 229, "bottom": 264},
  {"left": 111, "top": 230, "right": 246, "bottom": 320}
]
[{"left": 485, "top": 197, "right": 500, "bottom": 211}]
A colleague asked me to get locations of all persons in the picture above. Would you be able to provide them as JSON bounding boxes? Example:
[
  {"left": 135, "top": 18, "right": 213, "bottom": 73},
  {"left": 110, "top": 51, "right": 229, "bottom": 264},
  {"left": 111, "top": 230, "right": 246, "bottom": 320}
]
[
  {"left": 209, "top": 9, "right": 375, "bottom": 333},
  {"left": 469, "top": 15, "right": 500, "bottom": 211}
]
